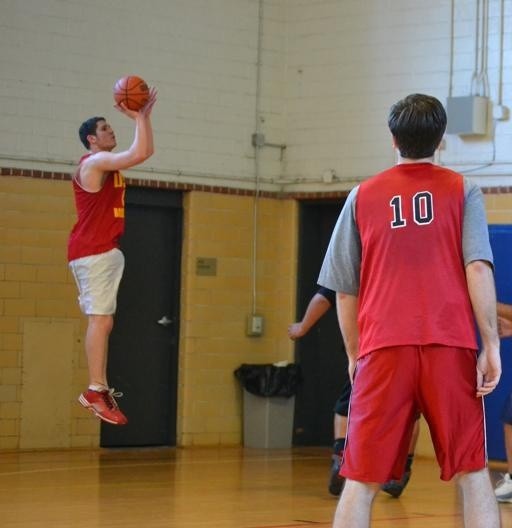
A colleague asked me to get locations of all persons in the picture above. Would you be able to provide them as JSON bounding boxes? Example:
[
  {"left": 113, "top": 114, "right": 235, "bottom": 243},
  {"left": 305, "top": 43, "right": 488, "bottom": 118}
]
[
  {"left": 492, "top": 300, "right": 512, "bottom": 505},
  {"left": 289, "top": 288, "right": 420, "bottom": 498},
  {"left": 316, "top": 95, "right": 502, "bottom": 528},
  {"left": 68, "top": 84, "right": 158, "bottom": 426}
]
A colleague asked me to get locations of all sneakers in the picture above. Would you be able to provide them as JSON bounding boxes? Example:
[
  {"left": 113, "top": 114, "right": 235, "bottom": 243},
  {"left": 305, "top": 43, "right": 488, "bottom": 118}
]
[
  {"left": 78, "top": 389, "right": 129, "bottom": 426},
  {"left": 328, "top": 454, "right": 345, "bottom": 496},
  {"left": 494, "top": 473, "right": 512, "bottom": 503},
  {"left": 381, "top": 472, "right": 409, "bottom": 498}
]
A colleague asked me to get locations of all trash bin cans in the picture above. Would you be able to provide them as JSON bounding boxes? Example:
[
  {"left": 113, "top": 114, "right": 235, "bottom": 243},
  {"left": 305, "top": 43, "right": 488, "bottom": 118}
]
[{"left": 242, "top": 364, "right": 301, "bottom": 449}]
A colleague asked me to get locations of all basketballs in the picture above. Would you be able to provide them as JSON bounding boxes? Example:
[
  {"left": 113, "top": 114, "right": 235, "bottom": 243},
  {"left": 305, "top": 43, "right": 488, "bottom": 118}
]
[{"left": 113, "top": 76, "right": 150, "bottom": 111}]
